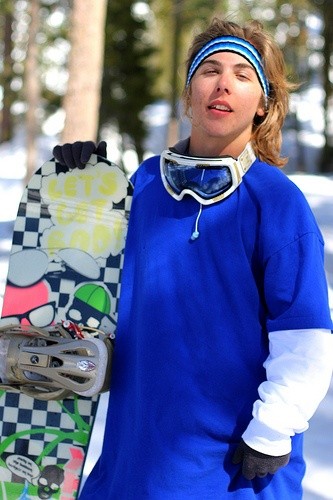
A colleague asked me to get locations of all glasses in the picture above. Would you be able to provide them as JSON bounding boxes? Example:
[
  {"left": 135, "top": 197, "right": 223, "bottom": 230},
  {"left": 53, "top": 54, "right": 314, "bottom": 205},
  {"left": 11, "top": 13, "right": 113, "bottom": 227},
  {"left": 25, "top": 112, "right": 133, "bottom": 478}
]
[{"left": 160, "top": 133, "right": 257, "bottom": 205}]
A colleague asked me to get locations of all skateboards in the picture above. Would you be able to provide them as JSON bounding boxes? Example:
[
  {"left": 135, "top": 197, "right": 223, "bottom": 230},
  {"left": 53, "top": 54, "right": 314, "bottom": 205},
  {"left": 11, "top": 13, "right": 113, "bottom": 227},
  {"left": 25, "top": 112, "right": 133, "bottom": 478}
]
[{"left": 0, "top": 154, "right": 133, "bottom": 499}]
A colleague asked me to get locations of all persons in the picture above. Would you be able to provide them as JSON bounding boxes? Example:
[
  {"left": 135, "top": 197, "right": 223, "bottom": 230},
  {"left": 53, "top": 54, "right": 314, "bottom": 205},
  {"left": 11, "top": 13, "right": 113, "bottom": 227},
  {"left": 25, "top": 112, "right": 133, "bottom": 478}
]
[{"left": 52, "top": 17, "right": 332, "bottom": 500}]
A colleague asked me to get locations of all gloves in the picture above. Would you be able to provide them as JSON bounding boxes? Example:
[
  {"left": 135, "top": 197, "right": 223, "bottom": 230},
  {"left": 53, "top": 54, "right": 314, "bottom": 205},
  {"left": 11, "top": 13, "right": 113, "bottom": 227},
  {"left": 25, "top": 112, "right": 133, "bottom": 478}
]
[
  {"left": 52, "top": 141, "right": 107, "bottom": 170},
  {"left": 229, "top": 440, "right": 291, "bottom": 480}
]
